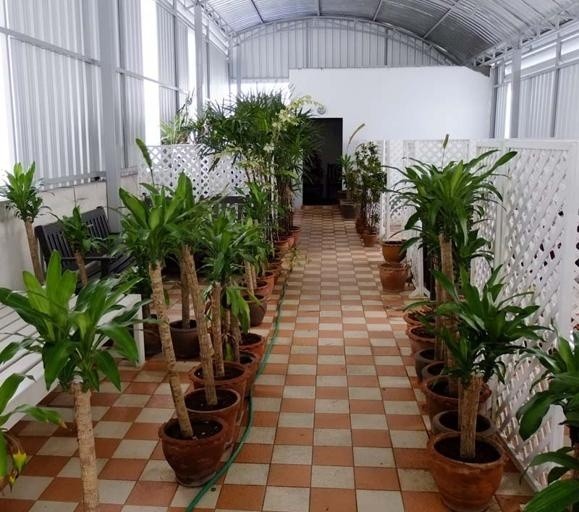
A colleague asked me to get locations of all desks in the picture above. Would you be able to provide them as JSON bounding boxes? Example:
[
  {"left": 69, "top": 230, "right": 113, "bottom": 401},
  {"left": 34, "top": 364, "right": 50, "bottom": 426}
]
[{"left": 0, "top": 290, "right": 146, "bottom": 433}]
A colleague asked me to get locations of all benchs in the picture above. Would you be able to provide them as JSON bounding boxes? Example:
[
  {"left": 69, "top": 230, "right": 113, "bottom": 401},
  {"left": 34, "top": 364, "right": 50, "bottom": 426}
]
[{"left": 35, "top": 204, "right": 138, "bottom": 294}]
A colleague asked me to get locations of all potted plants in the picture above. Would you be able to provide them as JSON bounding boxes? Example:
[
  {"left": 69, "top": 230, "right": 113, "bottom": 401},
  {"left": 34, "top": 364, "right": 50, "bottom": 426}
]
[{"left": 335, "top": 121, "right": 555, "bottom": 512}]
